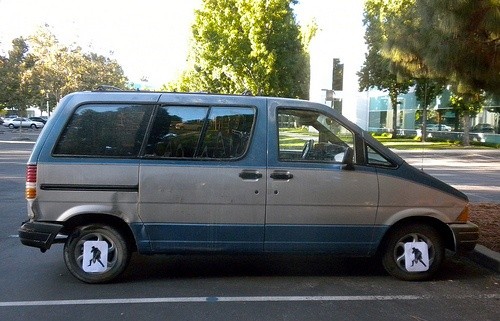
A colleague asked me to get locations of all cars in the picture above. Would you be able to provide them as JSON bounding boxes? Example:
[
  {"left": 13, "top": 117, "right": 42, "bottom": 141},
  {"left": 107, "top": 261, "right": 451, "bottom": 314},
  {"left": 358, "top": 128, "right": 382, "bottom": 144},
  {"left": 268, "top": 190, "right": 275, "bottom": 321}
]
[
  {"left": 3, "top": 114, "right": 47, "bottom": 129},
  {"left": 426, "top": 123, "right": 495, "bottom": 133}
]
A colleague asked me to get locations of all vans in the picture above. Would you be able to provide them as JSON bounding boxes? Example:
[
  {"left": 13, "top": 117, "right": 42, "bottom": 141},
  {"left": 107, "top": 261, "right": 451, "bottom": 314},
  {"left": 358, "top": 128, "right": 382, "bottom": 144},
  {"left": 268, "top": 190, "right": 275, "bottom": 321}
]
[{"left": 18, "top": 92, "right": 481, "bottom": 284}]
